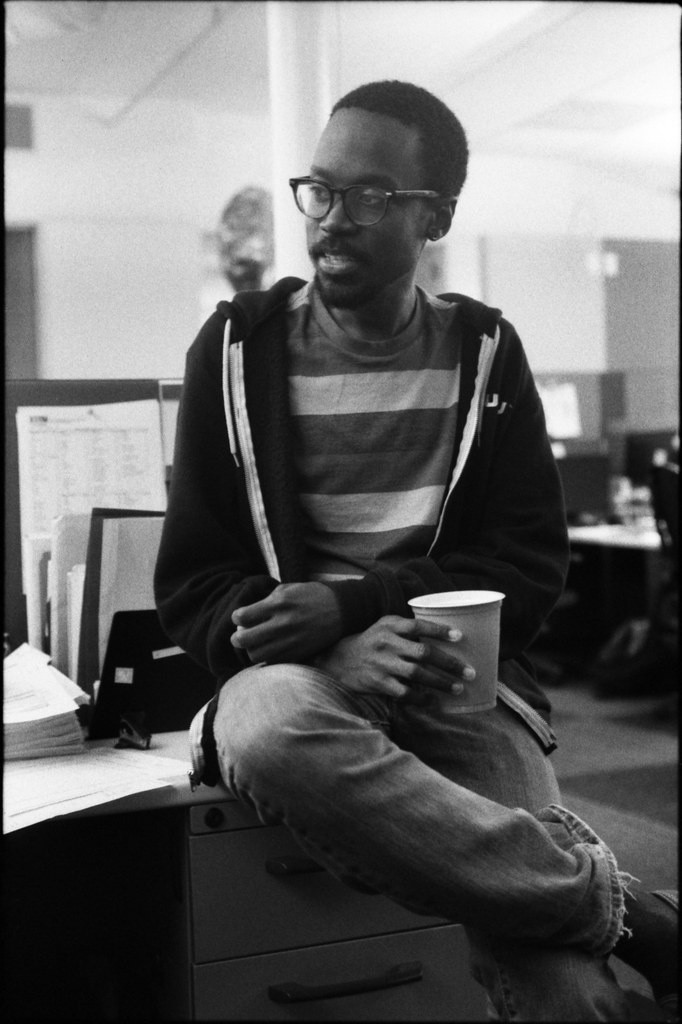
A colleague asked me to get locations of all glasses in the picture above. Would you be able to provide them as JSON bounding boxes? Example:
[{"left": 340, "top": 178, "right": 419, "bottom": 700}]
[{"left": 289, "top": 172, "right": 445, "bottom": 229}]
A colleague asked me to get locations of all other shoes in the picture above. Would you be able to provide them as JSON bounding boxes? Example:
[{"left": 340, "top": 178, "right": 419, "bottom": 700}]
[{"left": 648, "top": 887, "right": 681, "bottom": 1022}]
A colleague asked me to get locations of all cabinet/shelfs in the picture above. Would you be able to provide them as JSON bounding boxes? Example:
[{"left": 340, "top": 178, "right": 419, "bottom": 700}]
[{"left": 168, "top": 802, "right": 472, "bottom": 1024}]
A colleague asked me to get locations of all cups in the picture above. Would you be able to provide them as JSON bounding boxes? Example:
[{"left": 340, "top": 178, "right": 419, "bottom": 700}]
[{"left": 407, "top": 590, "right": 507, "bottom": 715}]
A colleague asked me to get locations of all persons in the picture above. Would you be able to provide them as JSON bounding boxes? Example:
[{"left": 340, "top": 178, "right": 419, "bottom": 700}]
[{"left": 154, "top": 82, "right": 681, "bottom": 1024}]
